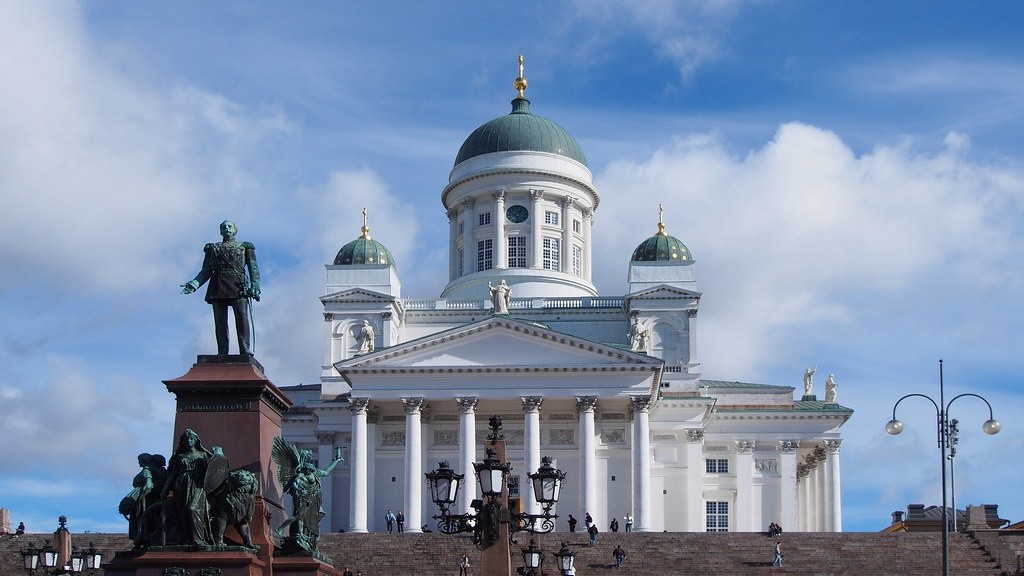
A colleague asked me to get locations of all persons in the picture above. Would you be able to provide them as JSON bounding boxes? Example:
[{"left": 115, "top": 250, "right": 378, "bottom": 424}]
[
  {"left": 568, "top": 514, "right": 577, "bottom": 532},
  {"left": 772, "top": 542, "right": 783, "bottom": 567},
  {"left": 609, "top": 518, "right": 618, "bottom": 532},
  {"left": 119, "top": 428, "right": 343, "bottom": 551},
  {"left": 460, "top": 553, "right": 470, "bottom": 576},
  {"left": 627, "top": 319, "right": 648, "bottom": 351},
  {"left": 343, "top": 567, "right": 363, "bottom": 576},
  {"left": 769, "top": 522, "right": 782, "bottom": 537},
  {"left": 803, "top": 366, "right": 817, "bottom": 395},
  {"left": 568, "top": 567, "right": 576, "bottom": 576},
  {"left": 179, "top": 220, "right": 261, "bottom": 356},
  {"left": 825, "top": 374, "right": 838, "bottom": 404},
  {"left": 384, "top": 509, "right": 404, "bottom": 534},
  {"left": 585, "top": 513, "right": 592, "bottom": 527},
  {"left": 613, "top": 545, "right": 626, "bottom": 569},
  {"left": 16, "top": 522, "right": 25, "bottom": 534},
  {"left": 356, "top": 320, "right": 374, "bottom": 355},
  {"left": 488, "top": 279, "right": 511, "bottom": 312},
  {"left": 588, "top": 524, "right": 598, "bottom": 546},
  {"left": 623, "top": 513, "right": 633, "bottom": 532}
]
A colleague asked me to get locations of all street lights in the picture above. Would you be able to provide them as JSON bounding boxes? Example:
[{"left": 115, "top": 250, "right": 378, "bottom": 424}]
[
  {"left": 424, "top": 413, "right": 569, "bottom": 576},
  {"left": 884, "top": 357, "right": 1002, "bottom": 576}
]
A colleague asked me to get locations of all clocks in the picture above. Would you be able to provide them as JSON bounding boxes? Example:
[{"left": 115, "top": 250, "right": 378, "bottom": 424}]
[{"left": 506, "top": 205, "right": 528, "bottom": 222}]
